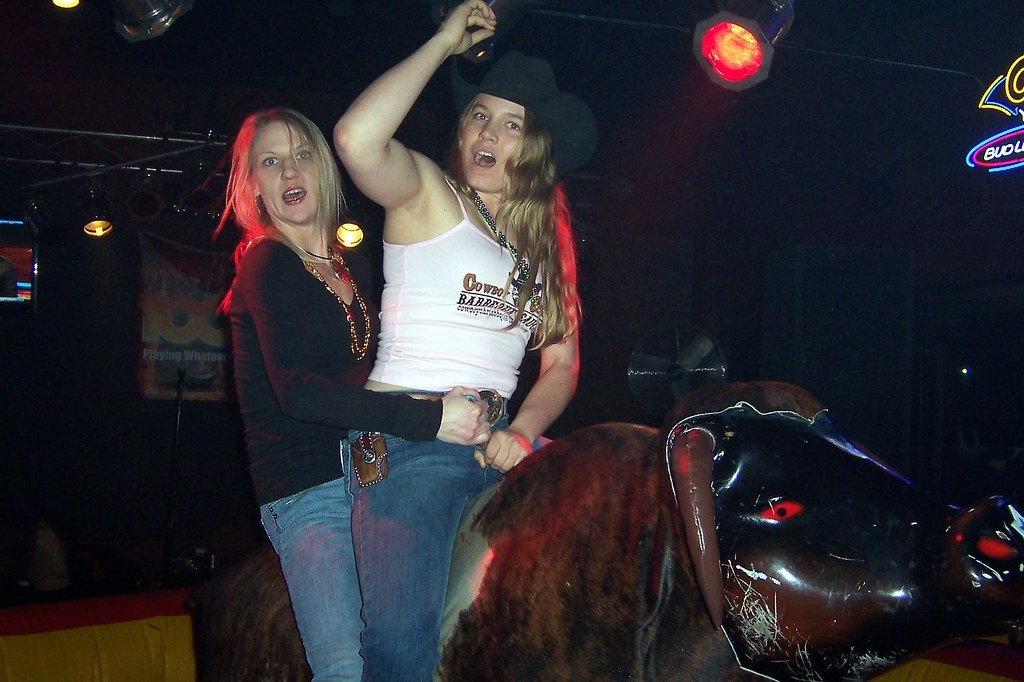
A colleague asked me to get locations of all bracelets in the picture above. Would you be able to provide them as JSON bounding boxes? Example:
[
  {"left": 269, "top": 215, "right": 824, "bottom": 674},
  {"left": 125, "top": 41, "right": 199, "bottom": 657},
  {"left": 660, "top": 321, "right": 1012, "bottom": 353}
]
[{"left": 511, "top": 430, "right": 534, "bottom": 453}]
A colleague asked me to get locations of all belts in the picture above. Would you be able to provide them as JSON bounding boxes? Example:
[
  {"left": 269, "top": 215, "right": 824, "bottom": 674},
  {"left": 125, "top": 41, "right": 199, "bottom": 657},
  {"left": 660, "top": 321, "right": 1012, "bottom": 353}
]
[{"left": 408, "top": 388, "right": 503, "bottom": 429}]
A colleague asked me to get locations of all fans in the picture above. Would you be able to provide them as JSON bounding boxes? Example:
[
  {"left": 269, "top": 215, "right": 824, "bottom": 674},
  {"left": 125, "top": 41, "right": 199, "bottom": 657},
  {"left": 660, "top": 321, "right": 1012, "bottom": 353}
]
[{"left": 620, "top": 322, "right": 730, "bottom": 424}]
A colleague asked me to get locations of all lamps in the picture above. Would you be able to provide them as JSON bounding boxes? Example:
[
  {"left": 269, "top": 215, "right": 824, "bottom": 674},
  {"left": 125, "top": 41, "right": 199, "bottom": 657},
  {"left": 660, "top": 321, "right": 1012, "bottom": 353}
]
[
  {"left": 691, "top": 0, "right": 795, "bottom": 91},
  {"left": 76, "top": 178, "right": 116, "bottom": 237},
  {"left": 109, "top": 0, "right": 192, "bottom": 42},
  {"left": 429, "top": 0, "right": 528, "bottom": 65}
]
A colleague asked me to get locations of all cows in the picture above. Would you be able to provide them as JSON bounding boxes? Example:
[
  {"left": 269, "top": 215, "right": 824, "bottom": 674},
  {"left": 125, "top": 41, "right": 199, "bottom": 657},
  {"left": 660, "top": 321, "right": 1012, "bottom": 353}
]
[{"left": 181, "top": 378, "right": 1024, "bottom": 682}]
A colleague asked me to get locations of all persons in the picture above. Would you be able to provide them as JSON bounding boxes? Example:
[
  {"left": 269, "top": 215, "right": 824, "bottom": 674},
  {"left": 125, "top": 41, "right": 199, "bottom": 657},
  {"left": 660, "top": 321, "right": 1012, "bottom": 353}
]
[
  {"left": 333, "top": 0, "right": 581, "bottom": 682},
  {"left": 0, "top": 255, "right": 18, "bottom": 297},
  {"left": 220, "top": 109, "right": 492, "bottom": 682}
]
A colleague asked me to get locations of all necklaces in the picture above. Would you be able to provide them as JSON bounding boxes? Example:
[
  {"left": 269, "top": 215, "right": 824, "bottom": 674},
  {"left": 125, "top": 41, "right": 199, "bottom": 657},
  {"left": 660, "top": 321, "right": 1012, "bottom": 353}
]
[
  {"left": 304, "top": 246, "right": 371, "bottom": 361},
  {"left": 470, "top": 192, "right": 543, "bottom": 313}
]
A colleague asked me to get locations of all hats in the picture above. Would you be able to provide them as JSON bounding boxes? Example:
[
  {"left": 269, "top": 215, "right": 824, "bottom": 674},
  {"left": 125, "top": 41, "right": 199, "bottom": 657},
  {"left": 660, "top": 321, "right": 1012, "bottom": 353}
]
[{"left": 449, "top": 51, "right": 598, "bottom": 174}]
